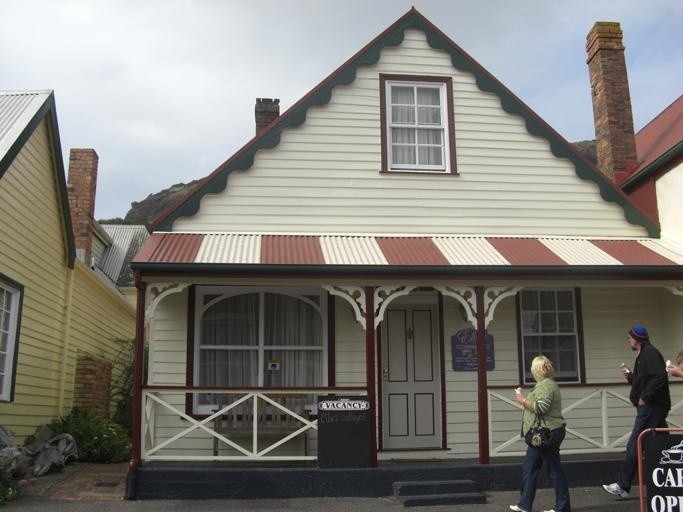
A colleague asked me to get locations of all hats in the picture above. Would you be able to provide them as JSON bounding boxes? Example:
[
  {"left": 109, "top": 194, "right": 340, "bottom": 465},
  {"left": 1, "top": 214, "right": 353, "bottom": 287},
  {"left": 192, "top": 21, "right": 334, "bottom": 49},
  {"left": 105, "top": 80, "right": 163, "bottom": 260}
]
[{"left": 629, "top": 326, "right": 649, "bottom": 342}]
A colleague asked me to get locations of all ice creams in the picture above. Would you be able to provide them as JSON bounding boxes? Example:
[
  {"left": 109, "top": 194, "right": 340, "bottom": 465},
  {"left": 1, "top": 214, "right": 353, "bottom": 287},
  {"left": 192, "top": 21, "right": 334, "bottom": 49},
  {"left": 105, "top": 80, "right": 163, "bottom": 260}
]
[
  {"left": 619, "top": 363, "right": 631, "bottom": 376},
  {"left": 514, "top": 387, "right": 524, "bottom": 396},
  {"left": 665, "top": 360, "right": 672, "bottom": 370}
]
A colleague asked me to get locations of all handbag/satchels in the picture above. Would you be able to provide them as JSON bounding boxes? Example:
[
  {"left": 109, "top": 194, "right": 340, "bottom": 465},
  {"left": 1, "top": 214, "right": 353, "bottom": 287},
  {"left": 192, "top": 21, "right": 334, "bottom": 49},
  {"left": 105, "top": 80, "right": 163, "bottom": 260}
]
[{"left": 525, "top": 427, "right": 552, "bottom": 448}]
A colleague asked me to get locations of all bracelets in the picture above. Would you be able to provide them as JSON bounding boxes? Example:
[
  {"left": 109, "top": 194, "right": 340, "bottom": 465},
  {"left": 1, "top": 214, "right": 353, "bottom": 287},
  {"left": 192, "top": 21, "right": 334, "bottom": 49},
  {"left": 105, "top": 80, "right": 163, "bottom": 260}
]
[{"left": 522, "top": 398, "right": 526, "bottom": 406}]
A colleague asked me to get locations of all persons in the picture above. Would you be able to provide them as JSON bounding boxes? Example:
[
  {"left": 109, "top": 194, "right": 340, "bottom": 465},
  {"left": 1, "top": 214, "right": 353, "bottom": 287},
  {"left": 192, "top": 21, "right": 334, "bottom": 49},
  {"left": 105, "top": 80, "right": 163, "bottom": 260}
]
[
  {"left": 509, "top": 354, "right": 570, "bottom": 512},
  {"left": 667, "top": 349, "right": 683, "bottom": 377},
  {"left": 602, "top": 323, "right": 672, "bottom": 500}
]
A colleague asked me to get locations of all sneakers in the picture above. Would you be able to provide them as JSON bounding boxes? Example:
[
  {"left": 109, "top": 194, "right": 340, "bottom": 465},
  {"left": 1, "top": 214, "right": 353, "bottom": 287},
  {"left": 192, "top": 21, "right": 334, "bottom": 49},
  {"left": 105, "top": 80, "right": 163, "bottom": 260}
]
[
  {"left": 510, "top": 505, "right": 527, "bottom": 512},
  {"left": 602, "top": 483, "right": 627, "bottom": 498},
  {"left": 544, "top": 510, "right": 555, "bottom": 512}
]
